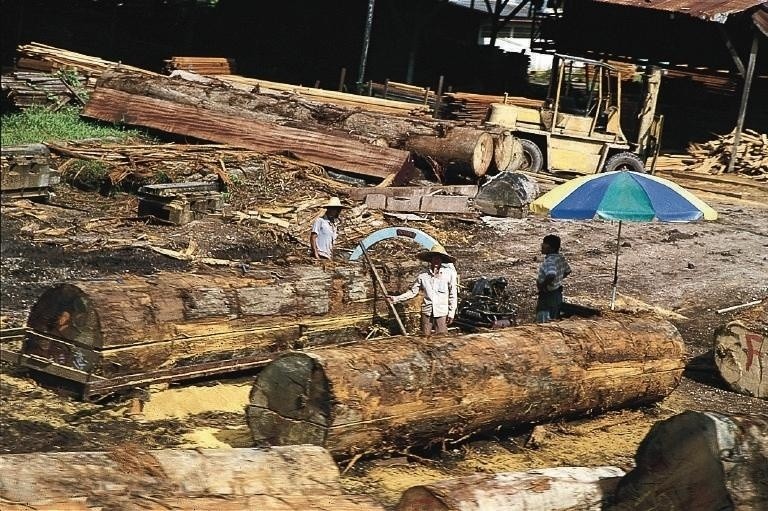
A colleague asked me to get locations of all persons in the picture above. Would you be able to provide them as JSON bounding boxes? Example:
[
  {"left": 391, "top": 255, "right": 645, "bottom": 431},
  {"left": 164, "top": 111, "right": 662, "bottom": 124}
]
[
  {"left": 308, "top": 196, "right": 347, "bottom": 262},
  {"left": 385, "top": 244, "right": 457, "bottom": 336},
  {"left": 535, "top": 234, "right": 566, "bottom": 323}
]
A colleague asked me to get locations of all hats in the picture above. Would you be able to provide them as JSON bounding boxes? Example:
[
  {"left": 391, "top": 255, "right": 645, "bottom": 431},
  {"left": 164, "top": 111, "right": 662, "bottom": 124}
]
[
  {"left": 417, "top": 245, "right": 456, "bottom": 263},
  {"left": 320, "top": 197, "right": 352, "bottom": 210}
]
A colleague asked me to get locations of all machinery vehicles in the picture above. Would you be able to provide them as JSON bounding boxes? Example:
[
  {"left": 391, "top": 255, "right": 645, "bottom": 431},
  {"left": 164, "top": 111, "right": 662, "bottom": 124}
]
[{"left": 481, "top": 52, "right": 647, "bottom": 175}]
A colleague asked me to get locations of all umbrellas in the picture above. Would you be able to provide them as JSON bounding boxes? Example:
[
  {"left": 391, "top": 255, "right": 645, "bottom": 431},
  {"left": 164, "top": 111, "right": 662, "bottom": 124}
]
[{"left": 529, "top": 167, "right": 721, "bottom": 313}]
